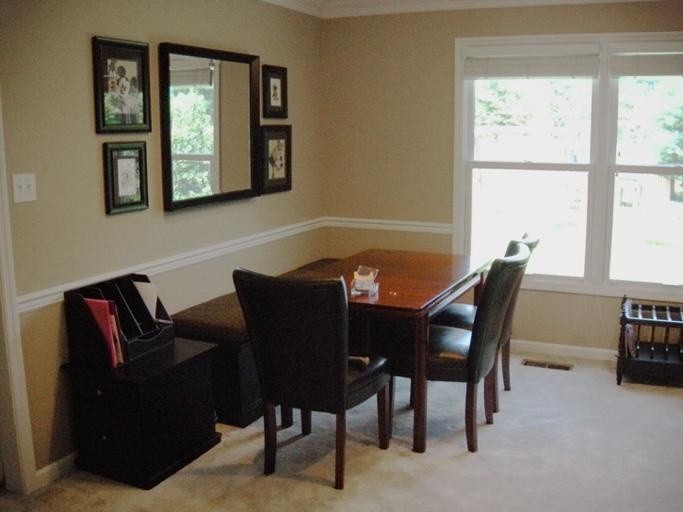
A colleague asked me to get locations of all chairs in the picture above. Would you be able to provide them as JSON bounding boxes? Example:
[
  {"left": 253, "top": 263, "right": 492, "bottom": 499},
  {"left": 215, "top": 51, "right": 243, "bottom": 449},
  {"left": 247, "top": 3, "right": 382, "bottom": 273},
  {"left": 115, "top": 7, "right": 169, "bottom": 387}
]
[
  {"left": 406, "top": 229, "right": 540, "bottom": 414},
  {"left": 229, "top": 266, "right": 396, "bottom": 491},
  {"left": 380, "top": 243, "right": 532, "bottom": 454}
]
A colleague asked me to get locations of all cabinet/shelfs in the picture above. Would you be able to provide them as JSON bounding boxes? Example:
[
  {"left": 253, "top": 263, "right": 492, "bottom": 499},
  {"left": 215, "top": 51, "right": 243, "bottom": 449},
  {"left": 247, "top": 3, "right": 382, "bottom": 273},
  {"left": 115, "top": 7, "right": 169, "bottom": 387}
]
[{"left": 59, "top": 337, "right": 226, "bottom": 490}]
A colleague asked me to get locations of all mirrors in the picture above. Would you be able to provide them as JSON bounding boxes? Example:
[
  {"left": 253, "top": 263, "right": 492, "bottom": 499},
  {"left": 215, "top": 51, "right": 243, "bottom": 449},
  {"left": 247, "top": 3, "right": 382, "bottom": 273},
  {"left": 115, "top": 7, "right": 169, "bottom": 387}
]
[{"left": 158, "top": 40, "right": 262, "bottom": 212}]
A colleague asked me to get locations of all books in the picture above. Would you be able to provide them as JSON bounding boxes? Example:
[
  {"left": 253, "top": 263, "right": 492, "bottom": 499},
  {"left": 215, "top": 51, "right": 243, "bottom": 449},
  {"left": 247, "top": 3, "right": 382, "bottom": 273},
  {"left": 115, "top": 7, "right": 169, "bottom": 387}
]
[{"left": 81, "top": 297, "right": 128, "bottom": 371}]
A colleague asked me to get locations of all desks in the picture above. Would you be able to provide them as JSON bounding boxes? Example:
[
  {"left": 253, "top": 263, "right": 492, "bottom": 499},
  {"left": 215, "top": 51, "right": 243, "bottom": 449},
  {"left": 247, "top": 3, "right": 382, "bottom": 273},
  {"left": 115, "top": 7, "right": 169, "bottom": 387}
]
[{"left": 279, "top": 245, "right": 495, "bottom": 453}]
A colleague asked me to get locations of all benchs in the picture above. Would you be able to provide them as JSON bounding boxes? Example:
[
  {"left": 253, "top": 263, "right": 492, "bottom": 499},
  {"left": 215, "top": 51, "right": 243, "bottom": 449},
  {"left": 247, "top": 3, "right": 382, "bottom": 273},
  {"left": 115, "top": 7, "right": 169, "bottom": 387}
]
[{"left": 169, "top": 256, "right": 342, "bottom": 428}]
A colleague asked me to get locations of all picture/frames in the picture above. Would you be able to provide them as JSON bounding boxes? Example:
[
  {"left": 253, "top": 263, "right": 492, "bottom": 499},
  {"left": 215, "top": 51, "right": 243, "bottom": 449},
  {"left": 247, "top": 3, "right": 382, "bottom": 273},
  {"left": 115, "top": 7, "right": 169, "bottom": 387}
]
[
  {"left": 91, "top": 34, "right": 153, "bottom": 133},
  {"left": 261, "top": 63, "right": 288, "bottom": 120},
  {"left": 103, "top": 141, "right": 149, "bottom": 216},
  {"left": 260, "top": 124, "right": 293, "bottom": 194}
]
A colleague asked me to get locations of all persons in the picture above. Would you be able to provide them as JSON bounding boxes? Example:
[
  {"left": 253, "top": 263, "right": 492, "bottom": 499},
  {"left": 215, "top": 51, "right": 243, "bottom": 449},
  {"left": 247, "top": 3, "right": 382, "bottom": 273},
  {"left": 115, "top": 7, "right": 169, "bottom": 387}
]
[
  {"left": 127, "top": 74, "right": 139, "bottom": 125},
  {"left": 115, "top": 65, "right": 129, "bottom": 125}
]
